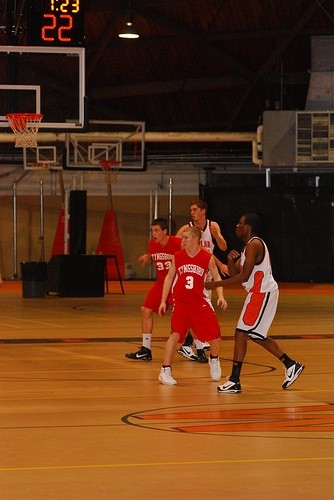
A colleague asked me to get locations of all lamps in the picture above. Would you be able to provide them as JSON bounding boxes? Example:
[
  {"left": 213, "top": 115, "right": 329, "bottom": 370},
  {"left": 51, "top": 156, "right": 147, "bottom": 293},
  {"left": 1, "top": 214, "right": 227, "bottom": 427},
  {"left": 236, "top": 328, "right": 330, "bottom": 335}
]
[{"left": 119, "top": 12, "right": 140, "bottom": 39}]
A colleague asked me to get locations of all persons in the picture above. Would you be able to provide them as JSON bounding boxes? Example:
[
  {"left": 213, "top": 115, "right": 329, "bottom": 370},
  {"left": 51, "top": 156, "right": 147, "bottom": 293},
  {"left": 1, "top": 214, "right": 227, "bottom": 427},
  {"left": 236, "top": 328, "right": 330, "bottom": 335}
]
[
  {"left": 158, "top": 226, "right": 228, "bottom": 386},
  {"left": 204, "top": 212, "right": 306, "bottom": 394},
  {"left": 176, "top": 199, "right": 227, "bottom": 361},
  {"left": 125, "top": 218, "right": 208, "bottom": 363}
]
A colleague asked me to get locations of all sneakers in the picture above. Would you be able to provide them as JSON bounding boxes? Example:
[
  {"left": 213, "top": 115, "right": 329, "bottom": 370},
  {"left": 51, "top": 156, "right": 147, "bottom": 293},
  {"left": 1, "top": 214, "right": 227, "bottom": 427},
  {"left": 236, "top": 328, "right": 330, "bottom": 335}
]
[
  {"left": 158, "top": 367, "right": 177, "bottom": 384},
  {"left": 217, "top": 379, "right": 242, "bottom": 393},
  {"left": 209, "top": 356, "right": 221, "bottom": 381},
  {"left": 125, "top": 347, "right": 152, "bottom": 360},
  {"left": 194, "top": 340, "right": 210, "bottom": 350},
  {"left": 177, "top": 346, "right": 198, "bottom": 360},
  {"left": 282, "top": 361, "right": 305, "bottom": 390},
  {"left": 197, "top": 349, "right": 209, "bottom": 362}
]
